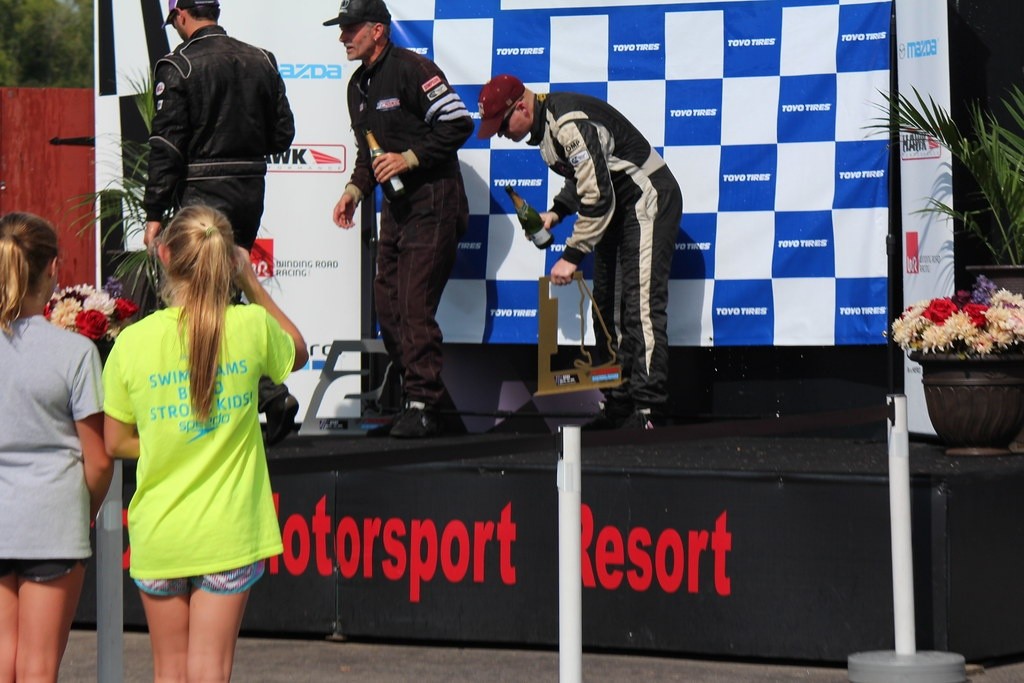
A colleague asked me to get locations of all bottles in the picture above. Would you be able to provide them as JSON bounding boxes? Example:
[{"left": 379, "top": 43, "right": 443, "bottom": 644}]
[
  {"left": 504, "top": 185, "right": 555, "bottom": 249},
  {"left": 361, "top": 125, "right": 407, "bottom": 203}
]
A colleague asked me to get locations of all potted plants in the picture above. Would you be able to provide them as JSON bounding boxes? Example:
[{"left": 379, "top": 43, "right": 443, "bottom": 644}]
[{"left": 861, "top": 80, "right": 1023, "bottom": 298}]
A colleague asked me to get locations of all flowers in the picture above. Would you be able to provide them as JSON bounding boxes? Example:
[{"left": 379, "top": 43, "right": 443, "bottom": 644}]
[
  {"left": 43, "top": 278, "right": 139, "bottom": 342},
  {"left": 892, "top": 274, "right": 1024, "bottom": 360}
]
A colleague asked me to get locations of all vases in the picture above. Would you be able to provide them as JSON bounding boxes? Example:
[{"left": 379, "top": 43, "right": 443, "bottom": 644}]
[{"left": 907, "top": 345, "right": 1023, "bottom": 456}]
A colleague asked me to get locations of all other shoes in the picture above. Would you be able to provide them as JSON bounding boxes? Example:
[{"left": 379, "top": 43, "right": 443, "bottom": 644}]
[
  {"left": 265, "top": 392, "right": 299, "bottom": 448},
  {"left": 582, "top": 407, "right": 667, "bottom": 430},
  {"left": 367, "top": 398, "right": 442, "bottom": 437}
]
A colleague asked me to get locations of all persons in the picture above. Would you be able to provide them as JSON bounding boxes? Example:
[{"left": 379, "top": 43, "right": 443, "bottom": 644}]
[
  {"left": 476, "top": 75, "right": 682, "bottom": 432},
  {"left": 323, "top": 0, "right": 476, "bottom": 437},
  {"left": 100, "top": 203, "right": 312, "bottom": 683},
  {"left": 0, "top": 214, "right": 114, "bottom": 683},
  {"left": 142, "top": 0, "right": 300, "bottom": 449}
]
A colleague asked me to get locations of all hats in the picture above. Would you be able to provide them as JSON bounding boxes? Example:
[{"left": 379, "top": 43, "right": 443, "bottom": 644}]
[
  {"left": 161, "top": 0, "right": 220, "bottom": 29},
  {"left": 323, "top": 0, "right": 391, "bottom": 26},
  {"left": 477, "top": 75, "right": 525, "bottom": 139}
]
